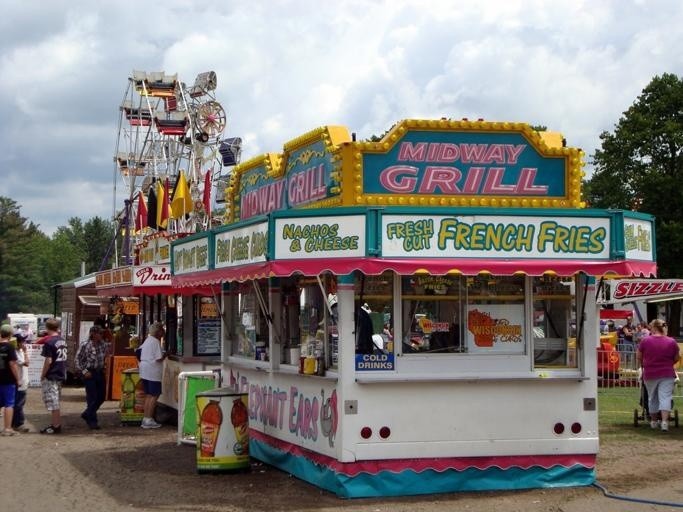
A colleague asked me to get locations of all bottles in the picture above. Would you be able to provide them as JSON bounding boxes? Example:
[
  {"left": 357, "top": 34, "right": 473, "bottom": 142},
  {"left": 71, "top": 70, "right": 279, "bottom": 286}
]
[
  {"left": 315, "top": 349, "right": 324, "bottom": 377},
  {"left": 301, "top": 354, "right": 307, "bottom": 374},
  {"left": 304, "top": 355, "right": 316, "bottom": 374}
]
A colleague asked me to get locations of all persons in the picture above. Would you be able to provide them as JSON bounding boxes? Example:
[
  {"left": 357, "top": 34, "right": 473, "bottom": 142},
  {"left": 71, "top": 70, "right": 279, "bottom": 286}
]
[
  {"left": 14, "top": 323, "right": 24, "bottom": 335},
  {"left": 1, "top": 324, "right": 22, "bottom": 436},
  {"left": 14, "top": 338, "right": 30, "bottom": 434},
  {"left": 640, "top": 321, "right": 649, "bottom": 338},
  {"left": 139, "top": 323, "right": 172, "bottom": 429},
  {"left": 22, "top": 323, "right": 34, "bottom": 342},
  {"left": 40, "top": 319, "right": 68, "bottom": 434},
  {"left": 604, "top": 320, "right": 625, "bottom": 339},
  {"left": 632, "top": 328, "right": 638, "bottom": 346},
  {"left": 35, "top": 331, "right": 50, "bottom": 344},
  {"left": 656, "top": 301, "right": 670, "bottom": 323},
  {"left": 637, "top": 319, "right": 681, "bottom": 432},
  {"left": 74, "top": 327, "right": 109, "bottom": 429},
  {"left": 621, "top": 317, "right": 636, "bottom": 369},
  {"left": 633, "top": 324, "right": 645, "bottom": 351}
]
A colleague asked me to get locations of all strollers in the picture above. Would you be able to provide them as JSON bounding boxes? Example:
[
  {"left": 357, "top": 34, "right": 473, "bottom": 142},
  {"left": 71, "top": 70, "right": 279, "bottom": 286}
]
[{"left": 633, "top": 362, "right": 678, "bottom": 428}]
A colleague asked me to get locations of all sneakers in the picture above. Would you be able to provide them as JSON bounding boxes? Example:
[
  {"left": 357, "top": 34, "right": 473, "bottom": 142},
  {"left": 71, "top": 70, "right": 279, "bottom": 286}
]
[
  {"left": 1, "top": 432, "right": 19, "bottom": 437},
  {"left": 649, "top": 421, "right": 655, "bottom": 427},
  {"left": 141, "top": 419, "right": 162, "bottom": 428},
  {"left": 16, "top": 425, "right": 29, "bottom": 433},
  {"left": 661, "top": 423, "right": 670, "bottom": 430},
  {"left": 39, "top": 424, "right": 62, "bottom": 436}
]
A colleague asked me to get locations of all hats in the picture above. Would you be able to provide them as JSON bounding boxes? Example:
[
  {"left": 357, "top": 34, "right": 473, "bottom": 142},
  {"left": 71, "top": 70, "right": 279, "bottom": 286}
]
[
  {"left": 12, "top": 333, "right": 29, "bottom": 342},
  {"left": 148, "top": 323, "right": 165, "bottom": 333},
  {"left": 90, "top": 327, "right": 106, "bottom": 334}
]
